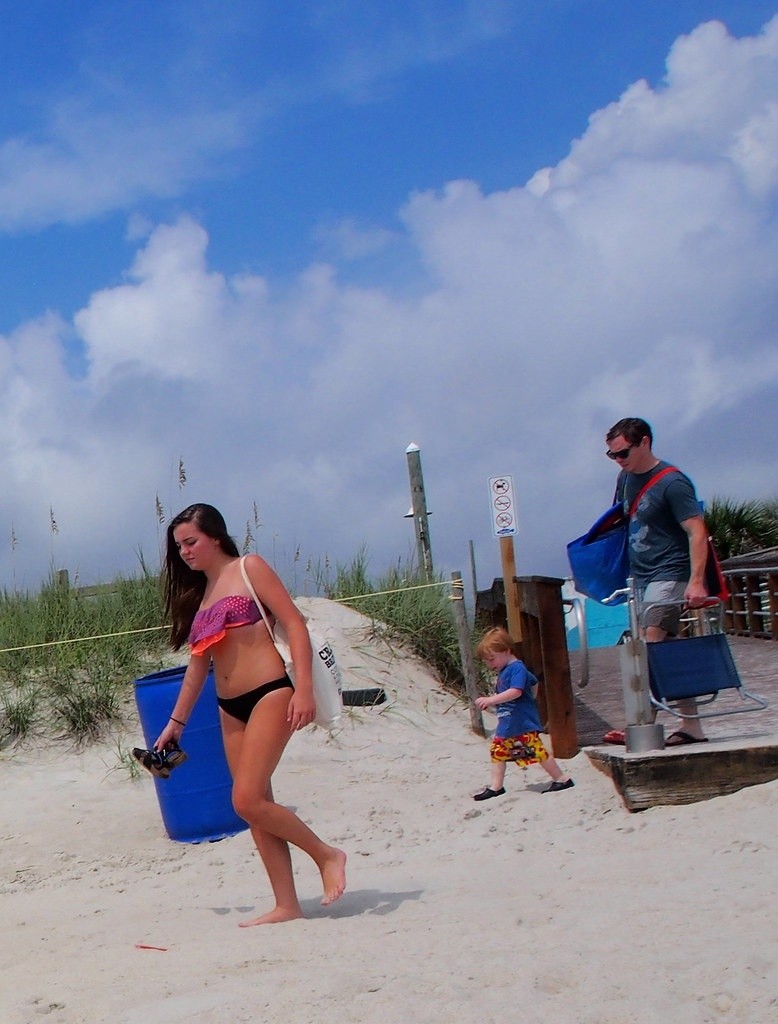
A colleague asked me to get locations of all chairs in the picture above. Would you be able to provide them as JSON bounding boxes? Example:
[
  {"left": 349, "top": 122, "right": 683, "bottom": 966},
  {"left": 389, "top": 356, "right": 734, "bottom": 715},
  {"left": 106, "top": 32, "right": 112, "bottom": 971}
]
[{"left": 643, "top": 597, "right": 767, "bottom": 720}]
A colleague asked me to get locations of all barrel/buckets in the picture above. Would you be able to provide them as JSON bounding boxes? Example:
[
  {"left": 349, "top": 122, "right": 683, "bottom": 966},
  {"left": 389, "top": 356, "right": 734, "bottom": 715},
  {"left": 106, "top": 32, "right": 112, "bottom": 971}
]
[{"left": 133, "top": 660, "right": 250, "bottom": 845}]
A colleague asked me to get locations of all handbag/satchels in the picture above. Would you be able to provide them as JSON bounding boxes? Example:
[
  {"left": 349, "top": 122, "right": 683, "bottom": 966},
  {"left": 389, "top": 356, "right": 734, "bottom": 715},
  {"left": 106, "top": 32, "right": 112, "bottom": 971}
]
[
  {"left": 240, "top": 553, "right": 344, "bottom": 730},
  {"left": 704, "top": 536, "right": 729, "bottom": 602}
]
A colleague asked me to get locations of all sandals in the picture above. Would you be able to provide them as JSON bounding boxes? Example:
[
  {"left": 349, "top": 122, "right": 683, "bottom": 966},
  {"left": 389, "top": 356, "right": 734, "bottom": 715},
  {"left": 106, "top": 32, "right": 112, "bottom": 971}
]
[
  {"left": 131, "top": 747, "right": 171, "bottom": 779},
  {"left": 154, "top": 738, "right": 188, "bottom": 770}
]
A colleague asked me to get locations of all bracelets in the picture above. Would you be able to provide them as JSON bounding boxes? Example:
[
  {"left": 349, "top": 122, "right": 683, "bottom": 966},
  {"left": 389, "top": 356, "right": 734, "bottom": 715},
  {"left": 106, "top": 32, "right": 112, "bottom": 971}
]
[{"left": 169, "top": 716, "right": 186, "bottom": 726}]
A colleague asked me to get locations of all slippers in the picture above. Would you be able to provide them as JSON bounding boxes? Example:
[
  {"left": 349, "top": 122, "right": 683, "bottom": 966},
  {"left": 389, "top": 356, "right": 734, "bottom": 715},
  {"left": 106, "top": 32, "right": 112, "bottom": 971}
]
[
  {"left": 664, "top": 731, "right": 709, "bottom": 747},
  {"left": 542, "top": 778, "right": 574, "bottom": 794},
  {"left": 474, "top": 787, "right": 505, "bottom": 801}
]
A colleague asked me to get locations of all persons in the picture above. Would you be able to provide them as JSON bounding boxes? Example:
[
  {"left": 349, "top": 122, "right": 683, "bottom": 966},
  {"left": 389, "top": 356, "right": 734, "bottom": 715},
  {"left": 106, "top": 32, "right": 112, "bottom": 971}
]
[
  {"left": 152, "top": 503, "right": 347, "bottom": 927},
  {"left": 474, "top": 626, "right": 575, "bottom": 801},
  {"left": 601, "top": 417, "right": 709, "bottom": 747}
]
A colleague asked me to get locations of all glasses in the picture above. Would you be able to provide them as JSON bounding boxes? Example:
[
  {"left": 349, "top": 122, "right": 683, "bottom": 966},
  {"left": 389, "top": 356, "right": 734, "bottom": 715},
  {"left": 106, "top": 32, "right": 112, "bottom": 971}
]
[{"left": 606, "top": 443, "right": 635, "bottom": 460}]
